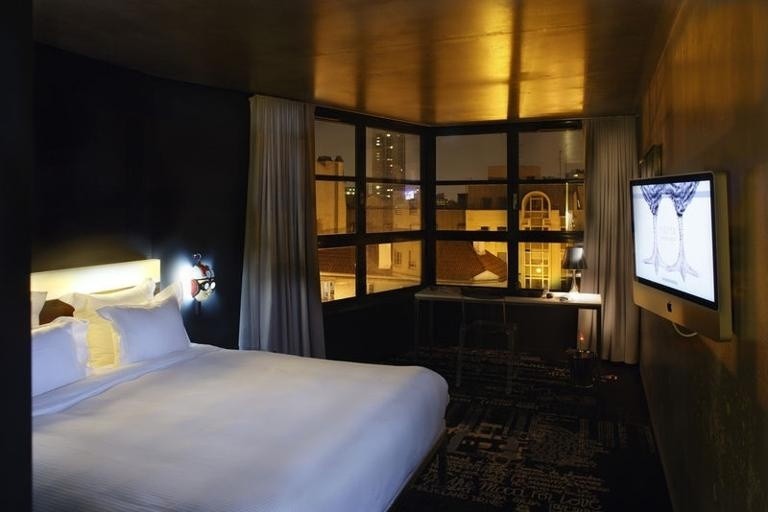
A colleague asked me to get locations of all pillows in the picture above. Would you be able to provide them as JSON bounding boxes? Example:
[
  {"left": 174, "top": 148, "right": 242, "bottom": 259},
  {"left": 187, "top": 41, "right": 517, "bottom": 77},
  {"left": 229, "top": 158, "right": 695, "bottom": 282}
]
[{"left": 28, "top": 277, "right": 192, "bottom": 397}]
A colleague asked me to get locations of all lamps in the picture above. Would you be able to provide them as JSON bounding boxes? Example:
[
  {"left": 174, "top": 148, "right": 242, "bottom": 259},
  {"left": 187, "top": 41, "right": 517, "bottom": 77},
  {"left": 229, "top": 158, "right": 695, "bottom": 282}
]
[{"left": 559, "top": 247, "right": 587, "bottom": 296}]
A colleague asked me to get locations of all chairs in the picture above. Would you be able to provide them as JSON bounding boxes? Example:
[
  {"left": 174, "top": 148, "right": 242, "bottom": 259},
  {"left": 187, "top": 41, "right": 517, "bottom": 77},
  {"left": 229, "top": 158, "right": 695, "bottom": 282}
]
[{"left": 454, "top": 287, "right": 519, "bottom": 395}]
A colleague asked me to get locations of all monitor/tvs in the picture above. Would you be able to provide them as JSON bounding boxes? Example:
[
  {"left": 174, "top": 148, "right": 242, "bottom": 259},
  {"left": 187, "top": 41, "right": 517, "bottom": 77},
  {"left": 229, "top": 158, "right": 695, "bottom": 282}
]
[{"left": 627, "top": 169, "right": 733, "bottom": 342}]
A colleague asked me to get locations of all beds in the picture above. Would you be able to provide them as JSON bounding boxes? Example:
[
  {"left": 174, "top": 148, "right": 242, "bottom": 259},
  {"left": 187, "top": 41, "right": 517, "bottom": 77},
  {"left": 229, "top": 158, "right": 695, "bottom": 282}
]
[{"left": 29, "top": 258, "right": 451, "bottom": 511}]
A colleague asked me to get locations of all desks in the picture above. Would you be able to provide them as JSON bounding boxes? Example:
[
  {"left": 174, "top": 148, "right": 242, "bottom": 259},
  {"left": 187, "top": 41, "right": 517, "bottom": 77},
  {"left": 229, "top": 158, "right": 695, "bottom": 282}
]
[{"left": 413, "top": 284, "right": 602, "bottom": 369}]
